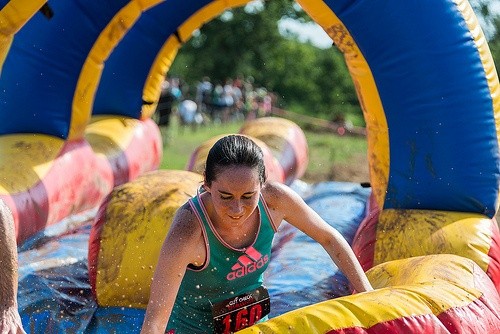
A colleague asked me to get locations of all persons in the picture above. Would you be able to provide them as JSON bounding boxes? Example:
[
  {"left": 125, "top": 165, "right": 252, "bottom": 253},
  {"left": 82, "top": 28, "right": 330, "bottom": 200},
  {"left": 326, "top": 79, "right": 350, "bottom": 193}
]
[
  {"left": 155, "top": 71, "right": 273, "bottom": 129},
  {"left": 139, "top": 134, "right": 376, "bottom": 333}
]
